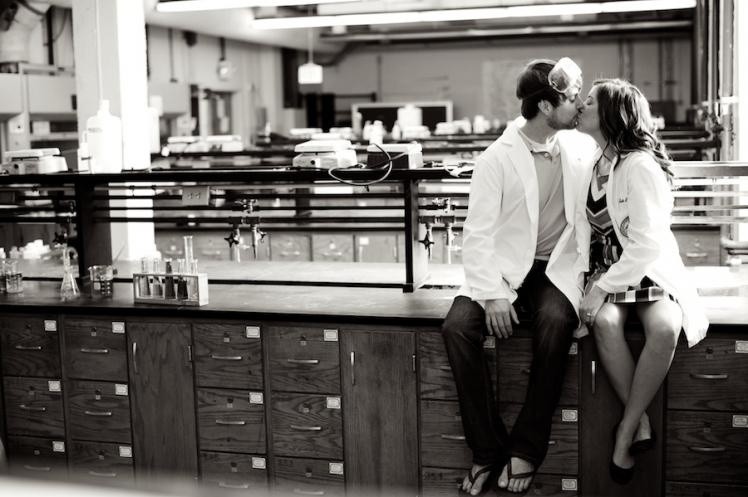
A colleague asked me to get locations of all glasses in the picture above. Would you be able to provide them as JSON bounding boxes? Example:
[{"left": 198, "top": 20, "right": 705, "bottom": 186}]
[{"left": 523, "top": 56, "right": 583, "bottom": 100}]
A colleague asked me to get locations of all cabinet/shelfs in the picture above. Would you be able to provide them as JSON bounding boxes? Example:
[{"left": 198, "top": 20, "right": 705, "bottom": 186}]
[{"left": 675, "top": 231, "right": 722, "bottom": 266}]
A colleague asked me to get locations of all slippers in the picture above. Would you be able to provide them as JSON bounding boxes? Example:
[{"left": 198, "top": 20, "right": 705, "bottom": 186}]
[
  {"left": 460, "top": 455, "right": 495, "bottom": 496},
  {"left": 495, "top": 458, "right": 537, "bottom": 493}
]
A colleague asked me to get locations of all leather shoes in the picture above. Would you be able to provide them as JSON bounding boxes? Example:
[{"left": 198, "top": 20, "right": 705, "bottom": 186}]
[{"left": 610, "top": 420, "right": 656, "bottom": 485}]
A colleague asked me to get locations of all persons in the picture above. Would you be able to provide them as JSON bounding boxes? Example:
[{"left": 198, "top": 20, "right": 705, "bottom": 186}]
[
  {"left": 574, "top": 76, "right": 712, "bottom": 486},
  {"left": 439, "top": 56, "right": 609, "bottom": 496}
]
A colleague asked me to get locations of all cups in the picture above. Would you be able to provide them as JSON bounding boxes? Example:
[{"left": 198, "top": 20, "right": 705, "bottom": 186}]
[
  {"left": 4, "top": 257, "right": 24, "bottom": 294},
  {"left": 88, "top": 265, "right": 114, "bottom": 300}
]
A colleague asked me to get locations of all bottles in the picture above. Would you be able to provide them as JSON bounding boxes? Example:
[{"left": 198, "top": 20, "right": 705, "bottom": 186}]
[
  {"left": 60, "top": 248, "right": 81, "bottom": 301},
  {"left": 138, "top": 257, "right": 199, "bottom": 301}
]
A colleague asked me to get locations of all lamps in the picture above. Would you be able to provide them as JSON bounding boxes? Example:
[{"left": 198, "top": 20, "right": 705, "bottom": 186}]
[{"left": 297, "top": 7, "right": 324, "bottom": 84}]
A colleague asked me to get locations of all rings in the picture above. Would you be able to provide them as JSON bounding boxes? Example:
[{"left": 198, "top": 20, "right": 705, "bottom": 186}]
[{"left": 585, "top": 313, "right": 590, "bottom": 317}]
